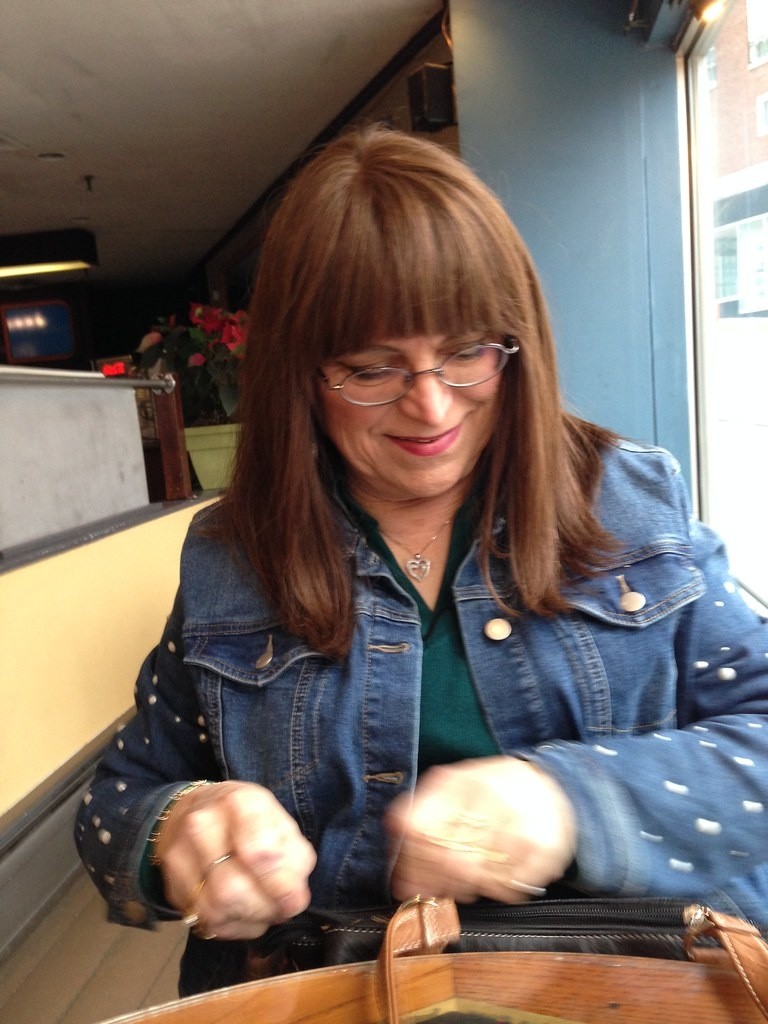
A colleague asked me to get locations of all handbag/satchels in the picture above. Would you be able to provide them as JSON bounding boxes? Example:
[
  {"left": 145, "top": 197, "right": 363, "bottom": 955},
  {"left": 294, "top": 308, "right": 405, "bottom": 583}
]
[{"left": 278, "top": 892, "right": 742, "bottom": 972}]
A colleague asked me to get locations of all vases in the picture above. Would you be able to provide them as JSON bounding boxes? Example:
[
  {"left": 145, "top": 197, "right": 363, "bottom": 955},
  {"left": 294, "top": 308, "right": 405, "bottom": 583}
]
[{"left": 185, "top": 423, "right": 241, "bottom": 489}]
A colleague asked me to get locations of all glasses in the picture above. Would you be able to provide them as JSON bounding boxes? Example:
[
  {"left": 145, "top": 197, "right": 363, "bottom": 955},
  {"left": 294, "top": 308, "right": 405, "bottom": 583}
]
[{"left": 315, "top": 337, "right": 519, "bottom": 407}]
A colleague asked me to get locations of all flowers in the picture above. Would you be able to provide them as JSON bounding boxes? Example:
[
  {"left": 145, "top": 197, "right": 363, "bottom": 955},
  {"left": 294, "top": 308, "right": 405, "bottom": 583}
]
[{"left": 135, "top": 305, "right": 249, "bottom": 425}]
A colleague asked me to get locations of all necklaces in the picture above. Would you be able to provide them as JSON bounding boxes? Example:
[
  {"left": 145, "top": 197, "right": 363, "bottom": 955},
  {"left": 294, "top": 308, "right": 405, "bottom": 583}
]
[{"left": 378, "top": 520, "right": 450, "bottom": 584}]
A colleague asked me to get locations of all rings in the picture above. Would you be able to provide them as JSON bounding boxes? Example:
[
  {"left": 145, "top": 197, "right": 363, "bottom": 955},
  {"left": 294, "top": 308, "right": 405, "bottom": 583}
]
[
  {"left": 502, "top": 878, "right": 546, "bottom": 898},
  {"left": 181, "top": 909, "right": 218, "bottom": 941},
  {"left": 207, "top": 854, "right": 233, "bottom": 873},
  {"left": 190, "top": 878, "right": 206, "bottom": 907}
]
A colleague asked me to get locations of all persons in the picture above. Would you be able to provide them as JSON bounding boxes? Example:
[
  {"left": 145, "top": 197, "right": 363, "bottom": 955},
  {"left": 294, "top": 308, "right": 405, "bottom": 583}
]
[{"left": 73, "top": 132, "right": 768, "bottom": 998}]
